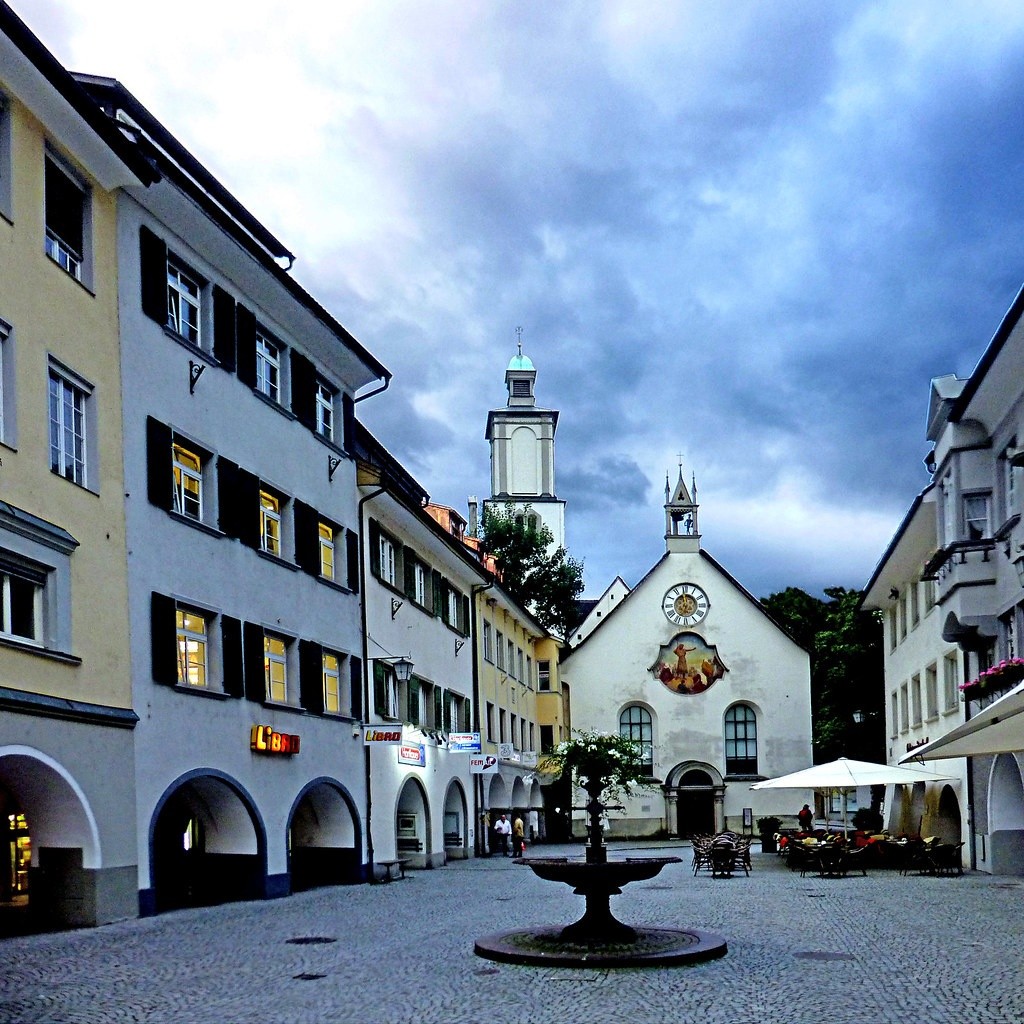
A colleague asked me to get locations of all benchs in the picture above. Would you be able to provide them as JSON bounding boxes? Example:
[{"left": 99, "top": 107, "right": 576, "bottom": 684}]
[
  {"left": 779, "top": 828, "right": 798, "bottom": 831},
  {"left": 377, "top": 859, "right": 412, "bottom": 882}
]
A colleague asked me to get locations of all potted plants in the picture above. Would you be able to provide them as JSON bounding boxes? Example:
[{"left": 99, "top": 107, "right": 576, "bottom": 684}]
[{"left": 755, "top": 816, "right": 783, "bottom": 853}]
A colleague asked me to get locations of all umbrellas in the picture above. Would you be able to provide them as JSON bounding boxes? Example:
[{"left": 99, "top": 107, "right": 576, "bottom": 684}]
[
  {"left": 749, "top": 758, "right": 961, "bottom": 841},
  {"left": 897, "top": 680, "right": 1024, "bottom": 765}
]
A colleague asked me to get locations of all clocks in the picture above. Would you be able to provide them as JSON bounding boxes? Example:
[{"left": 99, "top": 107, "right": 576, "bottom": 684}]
[{"left": 662, "top": 583, "right": 710, "bottom": 627}]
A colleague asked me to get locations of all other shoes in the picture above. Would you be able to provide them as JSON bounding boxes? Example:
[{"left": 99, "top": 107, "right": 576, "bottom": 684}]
[
  {"left": 517, "top": 854, "right": 523, "bottom": 858},
  {"left": 509, "top": 854, "right": 518, "bottom": 858}
]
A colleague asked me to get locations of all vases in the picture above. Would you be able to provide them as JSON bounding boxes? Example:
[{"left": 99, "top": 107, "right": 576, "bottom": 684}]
[{"left": 576, "top": 759, "right": 612, "bottom": 776}]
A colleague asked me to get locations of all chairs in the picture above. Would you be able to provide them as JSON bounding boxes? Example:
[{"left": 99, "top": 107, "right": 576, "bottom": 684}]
[
  {"left": 773, "top": 829, "right": 965, "bottom": 879},
  {"left": 692, "top": 833, "right": 753, "bottom": 879}
]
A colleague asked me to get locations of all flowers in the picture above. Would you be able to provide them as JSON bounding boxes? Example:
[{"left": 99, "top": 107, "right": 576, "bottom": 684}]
[
  {"left": 516, "top": 726, "right": 665, "bottom": 821},
  {"left": 958, "top": 658, "right": 1024, "bottom": 692}
]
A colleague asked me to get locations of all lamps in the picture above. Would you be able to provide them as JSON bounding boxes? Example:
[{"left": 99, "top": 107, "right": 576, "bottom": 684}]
[
  {"left": 888, "top": 588, "right": 899, "bottom": 600},
  {"left": 367, "top": 651, "right": 414, "bottom": 682}
]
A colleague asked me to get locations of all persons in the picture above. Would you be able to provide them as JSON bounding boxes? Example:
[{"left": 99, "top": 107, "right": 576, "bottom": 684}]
[
  {"left": 799, "top": 804, "right": 814, "bottom": 831},
  {"left": 511, "top": 814, "right": 524, "bottom": 858},
  {"left": 494, "top": 814, "right": 512, "bottom": 858}
]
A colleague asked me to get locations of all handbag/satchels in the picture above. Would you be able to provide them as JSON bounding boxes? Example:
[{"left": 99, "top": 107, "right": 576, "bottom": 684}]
[{"left": 521, "top": 842, "right": 526, "bottom": 851}]
